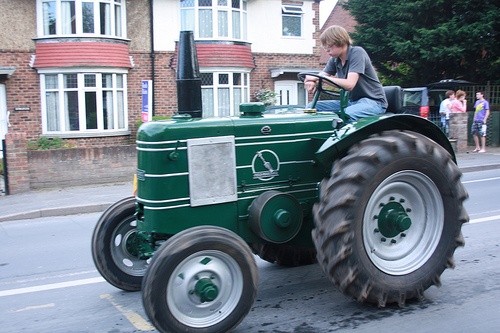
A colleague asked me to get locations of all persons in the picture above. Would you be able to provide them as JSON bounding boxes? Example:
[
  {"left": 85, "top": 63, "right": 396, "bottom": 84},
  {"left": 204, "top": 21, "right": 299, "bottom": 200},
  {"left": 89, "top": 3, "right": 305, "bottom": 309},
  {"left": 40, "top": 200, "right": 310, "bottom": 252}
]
[
  {"left": 440, "top": 91, "right": 456, "bottom": 131},
  {"left": 303, "top": 25, "right": 389, "bottom": 121},
  {"left": 449, "top": 90, "right": 467, "bottom": 113},
  {"left": 471, "top": 92, "right": 490, "bottom": 152}
]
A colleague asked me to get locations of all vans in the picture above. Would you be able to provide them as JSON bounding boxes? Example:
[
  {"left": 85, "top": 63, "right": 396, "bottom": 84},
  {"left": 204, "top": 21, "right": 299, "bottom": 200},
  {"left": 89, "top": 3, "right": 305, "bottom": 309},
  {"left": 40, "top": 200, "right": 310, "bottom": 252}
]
[{"left": 400, "top": 87, "right": 450, "bottom": 128}]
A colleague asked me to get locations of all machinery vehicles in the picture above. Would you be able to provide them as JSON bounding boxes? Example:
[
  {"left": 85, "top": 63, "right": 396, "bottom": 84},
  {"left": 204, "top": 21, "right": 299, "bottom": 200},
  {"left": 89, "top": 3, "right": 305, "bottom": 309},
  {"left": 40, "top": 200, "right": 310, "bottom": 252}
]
[{"left": 89, "top": 70, "right": 470, "bottom": 333}]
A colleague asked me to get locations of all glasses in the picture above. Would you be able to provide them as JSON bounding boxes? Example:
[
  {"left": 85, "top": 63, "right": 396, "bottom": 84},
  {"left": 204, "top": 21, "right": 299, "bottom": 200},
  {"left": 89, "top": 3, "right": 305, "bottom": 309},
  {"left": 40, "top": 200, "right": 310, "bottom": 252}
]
[{"left": 322, "top": 42, "right": 336, "bottom": 50}]
